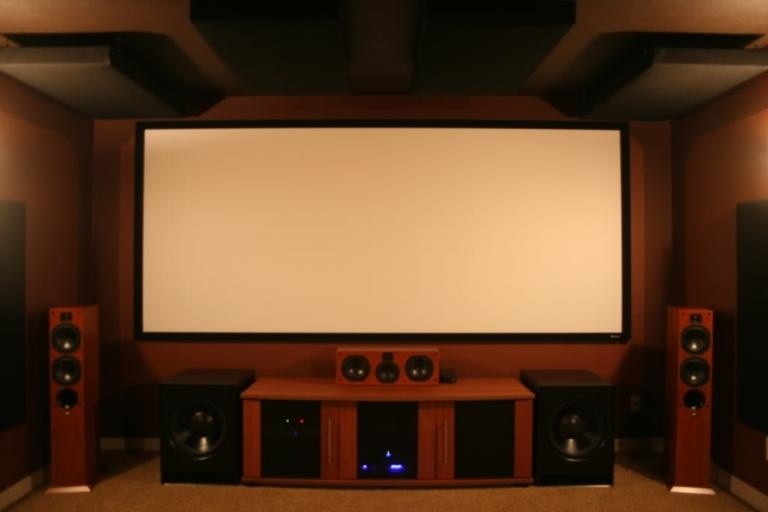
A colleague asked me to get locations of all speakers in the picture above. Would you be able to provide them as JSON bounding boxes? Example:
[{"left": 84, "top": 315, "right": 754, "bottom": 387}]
[
  {"left": 334, "top": 345, "right": 442, "bottom": 386},
  {"left": 47, "top": 304, "right": 101, "bottom": 493},
  {"left": 665, "top": 304, "right": 718, "bottom": 497},
  {"left": 518, "top": 369, "right": 616, "bottom": 489},
  {"left": 156, "top": 369, "right": 255, "bottom": 485}
]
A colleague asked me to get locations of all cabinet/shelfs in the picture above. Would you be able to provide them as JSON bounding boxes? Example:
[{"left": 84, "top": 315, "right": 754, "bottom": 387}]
[{"left": 243, "top": 373, "right": 535, "bottom": 490}]
